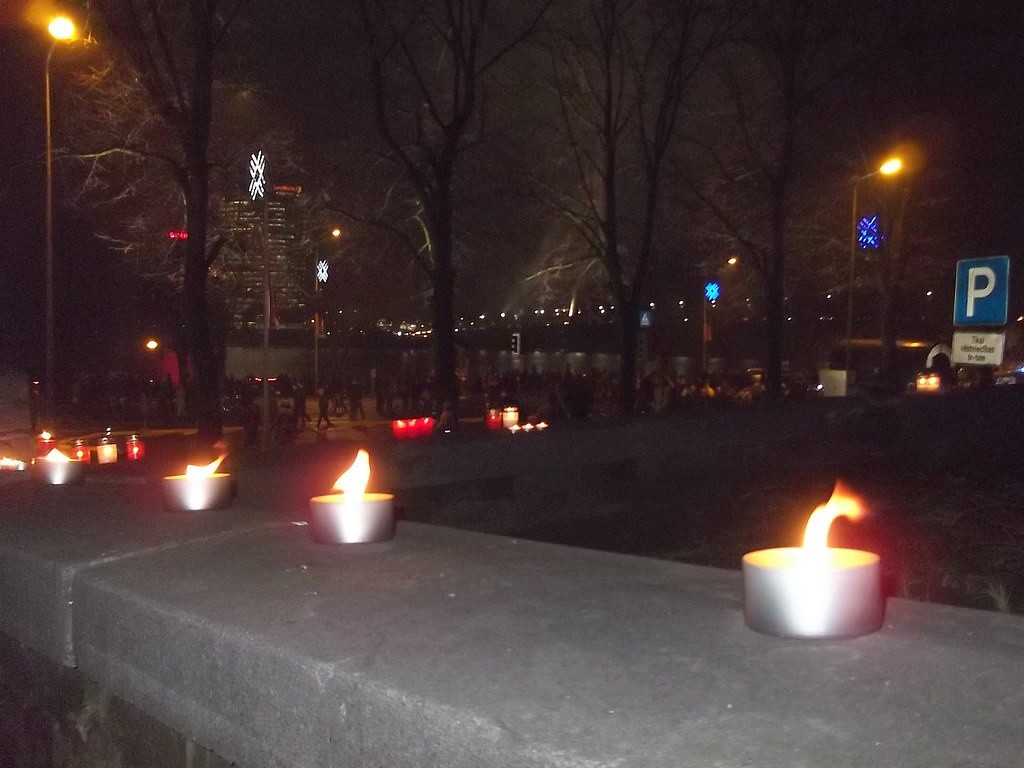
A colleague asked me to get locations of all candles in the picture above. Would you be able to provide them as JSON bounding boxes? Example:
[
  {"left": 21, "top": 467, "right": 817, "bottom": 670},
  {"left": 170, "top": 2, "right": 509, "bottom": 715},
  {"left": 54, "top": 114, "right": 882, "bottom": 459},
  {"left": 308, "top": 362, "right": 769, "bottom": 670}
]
[
  {"left": 503, "top": 407, "right": 518, "bottom": 427},
  {"left": 391, "top": 417, "right": 435, "bottom": 437},
  {"left": 161, "top": 451, "right": 231, "bottom": 511},
  {"left": 310, "top": 449, "right": 395, "bottom": 543},
  {"left": 740, "top": 485, "right": 882, "bottom": 637},
  {"left": 126, "top": 434, "right": 145, "bottom": 460},
  {"left": 95, "top": 435, "right": 118, "bottom": 464},
  {"left": 485, "top": 407, "right": 502, "bottom": 429},
  {"left": 35, "top": 448, "right": 85, "bottom": 485}
]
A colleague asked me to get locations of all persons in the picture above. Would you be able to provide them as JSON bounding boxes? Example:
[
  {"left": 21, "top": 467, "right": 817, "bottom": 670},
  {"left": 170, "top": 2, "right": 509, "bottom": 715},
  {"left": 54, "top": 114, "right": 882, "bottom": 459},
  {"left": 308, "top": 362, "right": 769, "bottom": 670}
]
[{"left": 29, "top": 363, "right": 809, "bottom": 434}]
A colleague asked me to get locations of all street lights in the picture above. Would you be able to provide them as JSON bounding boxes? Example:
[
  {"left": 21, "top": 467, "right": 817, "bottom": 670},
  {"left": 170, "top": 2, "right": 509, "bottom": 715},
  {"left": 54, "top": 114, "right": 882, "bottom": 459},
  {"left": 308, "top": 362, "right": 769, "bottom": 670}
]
[
  {"left": 43, "top": 12, "right": 74, "bottom": 409},
  {"left": 842, "top": 156, "right": 904, "bottom": 391}
]
